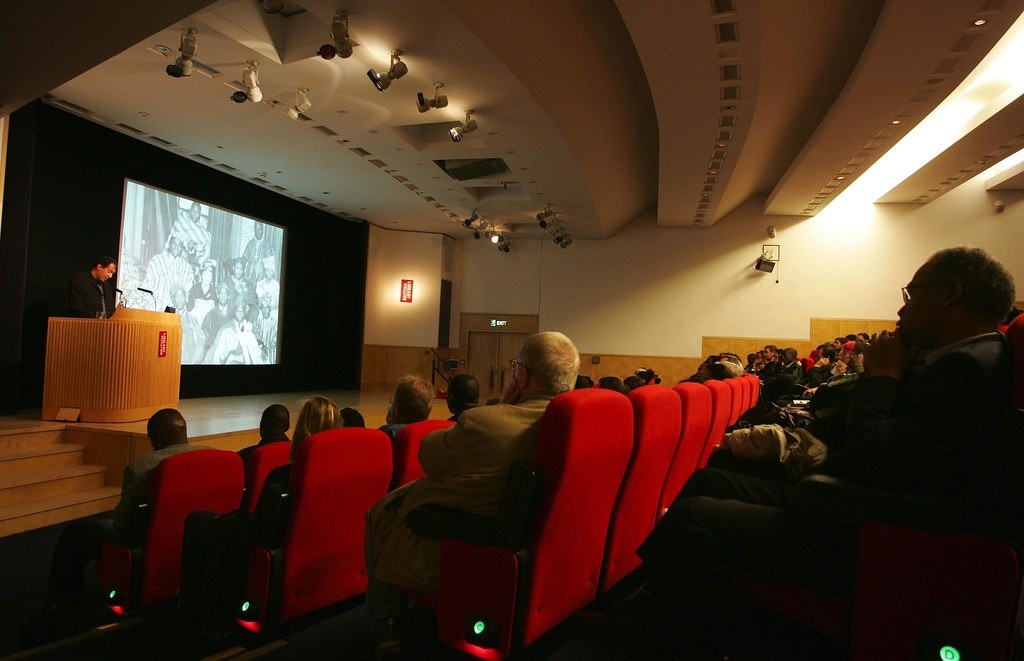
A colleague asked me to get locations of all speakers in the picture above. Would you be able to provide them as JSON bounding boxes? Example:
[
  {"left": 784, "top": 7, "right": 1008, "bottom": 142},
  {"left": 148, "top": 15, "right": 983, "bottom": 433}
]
[{"left": 755, "top": 260, "right": 775, "bottom": 273}]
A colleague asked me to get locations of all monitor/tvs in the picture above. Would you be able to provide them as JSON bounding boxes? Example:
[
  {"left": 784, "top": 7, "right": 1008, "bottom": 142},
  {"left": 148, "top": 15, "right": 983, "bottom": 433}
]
[{"left": 164, "top": 306, "right": 176, "bottom": 313}]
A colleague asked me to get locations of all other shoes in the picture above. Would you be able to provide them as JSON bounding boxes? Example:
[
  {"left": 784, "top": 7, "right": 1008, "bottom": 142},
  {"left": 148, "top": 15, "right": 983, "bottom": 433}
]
[{"left": 365, "top": 615, "right": 415, "bottom": 661}]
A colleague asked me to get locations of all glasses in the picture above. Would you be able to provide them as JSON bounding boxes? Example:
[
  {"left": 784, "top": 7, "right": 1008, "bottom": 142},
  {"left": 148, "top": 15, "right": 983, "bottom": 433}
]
[
  {"left": 387, "top": 395, "right": 400, "bottom": 406},
  {"left": 510, "top": 359, "right": 532, "bottom": 381},
  {"left": 756, "top": 355, "right": 763, "bottom": 359},
  {"left": 901, "top": 281, "right": 951, "bottom": 308}
]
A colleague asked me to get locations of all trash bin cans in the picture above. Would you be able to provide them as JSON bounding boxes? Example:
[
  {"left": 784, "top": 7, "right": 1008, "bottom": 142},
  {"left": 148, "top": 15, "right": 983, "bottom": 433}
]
[{"left": 437, "top": 388, "right": 447, "bottom": 399}]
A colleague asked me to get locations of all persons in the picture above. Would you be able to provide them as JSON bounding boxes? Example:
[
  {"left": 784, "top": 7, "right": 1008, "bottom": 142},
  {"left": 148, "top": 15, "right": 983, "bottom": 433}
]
[
  {"left": 70, "top": 256, "right": 117, "bottom": 318},
  {"left": 139, "top": 201, "right": 279, "bottom": 364},
  {"left": 115, "top": 248, "right": 1024, "bottom": 661}
]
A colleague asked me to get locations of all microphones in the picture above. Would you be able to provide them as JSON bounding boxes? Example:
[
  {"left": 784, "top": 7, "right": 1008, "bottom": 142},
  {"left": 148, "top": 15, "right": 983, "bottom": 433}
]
[
  {"left": 137, "top": 287, "right": 157, "bottom": 311},
  {"left": 111, "top": 286, "right": 124, "bottom": 306}
]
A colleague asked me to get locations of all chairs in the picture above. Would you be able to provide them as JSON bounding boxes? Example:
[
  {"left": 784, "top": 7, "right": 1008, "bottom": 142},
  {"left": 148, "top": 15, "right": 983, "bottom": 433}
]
[
  {"left": 98, "top": 371, "right": 761, "bottom": 661},
  {"left": 736, "top": 312, "right": 1024, "bottom": 661}
]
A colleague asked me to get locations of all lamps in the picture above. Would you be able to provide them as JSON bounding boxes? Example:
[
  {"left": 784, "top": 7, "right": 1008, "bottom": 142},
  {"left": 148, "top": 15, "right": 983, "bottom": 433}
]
[
  {"left": 261, "top": 0, "right": 284, "bottom": 14},
  {"left": 461, "top": 208, "right": 511, "bottom": 253},
  {"left": 754, "top": 250, "right": 775, "bottom": 273},
  {"left": 536, "top": 204, "right": 573, "bottom": 249},
  {"left": 366, "top": 49, "right": 407, "bottom": 92},
  {"left": 317, "top": 8, "right": 353, "bottom": 60},
  {"left": 166, "top": 28, "right": 199, "bottom": 78},
  {"left": 284, "top": 87, "right": 312, "bottom": 120},
  {"left": 230, "top": 61, "right": 263, "bottom": 103},
  {"left": 416, "top": 81, "right": 448, "bottom": 113},
  {"left": 447, "top": 109, "right": 478, "bottom": 143}
]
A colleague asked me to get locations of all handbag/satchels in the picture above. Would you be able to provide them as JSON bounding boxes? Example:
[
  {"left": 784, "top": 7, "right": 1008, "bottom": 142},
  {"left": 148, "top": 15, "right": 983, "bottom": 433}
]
[{"left": 727, "top": 422, "right": 800, "bottom": 465}]
